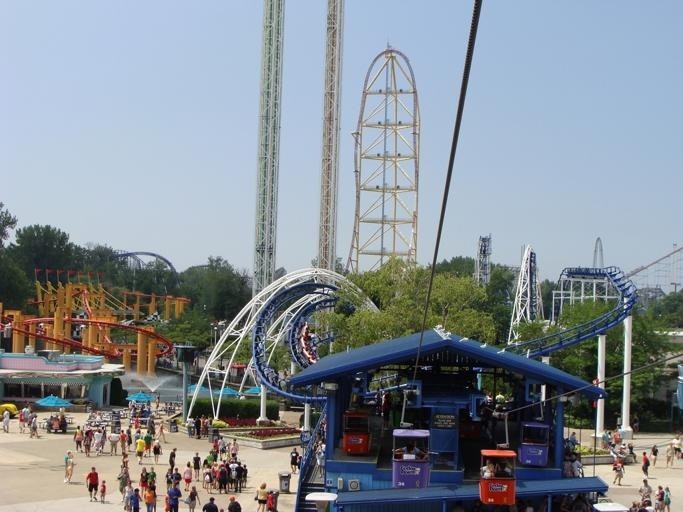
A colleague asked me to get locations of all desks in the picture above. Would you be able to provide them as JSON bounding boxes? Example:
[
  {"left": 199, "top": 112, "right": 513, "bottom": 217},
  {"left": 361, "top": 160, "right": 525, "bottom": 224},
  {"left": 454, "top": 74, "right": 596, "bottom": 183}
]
[{"left": 21, "top": 400, "right": 184, "bottom": 434}]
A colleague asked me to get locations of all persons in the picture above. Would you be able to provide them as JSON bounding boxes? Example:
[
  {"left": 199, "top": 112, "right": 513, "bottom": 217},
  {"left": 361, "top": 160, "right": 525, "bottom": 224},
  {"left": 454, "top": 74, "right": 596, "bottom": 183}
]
[
  {"left": 303, "top": 326, "right": 317, "bottom": 362},
  {"left": 1, "top": 391, "right": 325, "bottom": 511},
  {"left": 373, "top": 390, "right": 682, "bottom": 512}
]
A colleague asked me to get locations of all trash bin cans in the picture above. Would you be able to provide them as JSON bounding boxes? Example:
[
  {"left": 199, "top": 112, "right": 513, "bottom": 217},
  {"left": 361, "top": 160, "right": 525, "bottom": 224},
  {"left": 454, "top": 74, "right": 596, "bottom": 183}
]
[
  {"left": 208, "top": 428, "right": 219, "bottom": 443},
  {"left": 111, "top": 409, "right": 122, "bottom": 433},
  {"left": 169, "top": 419, "right": 177, "bottom": 433},
  {"left": 279, "top": 473, "right": 291, "bottom": 493}
]
